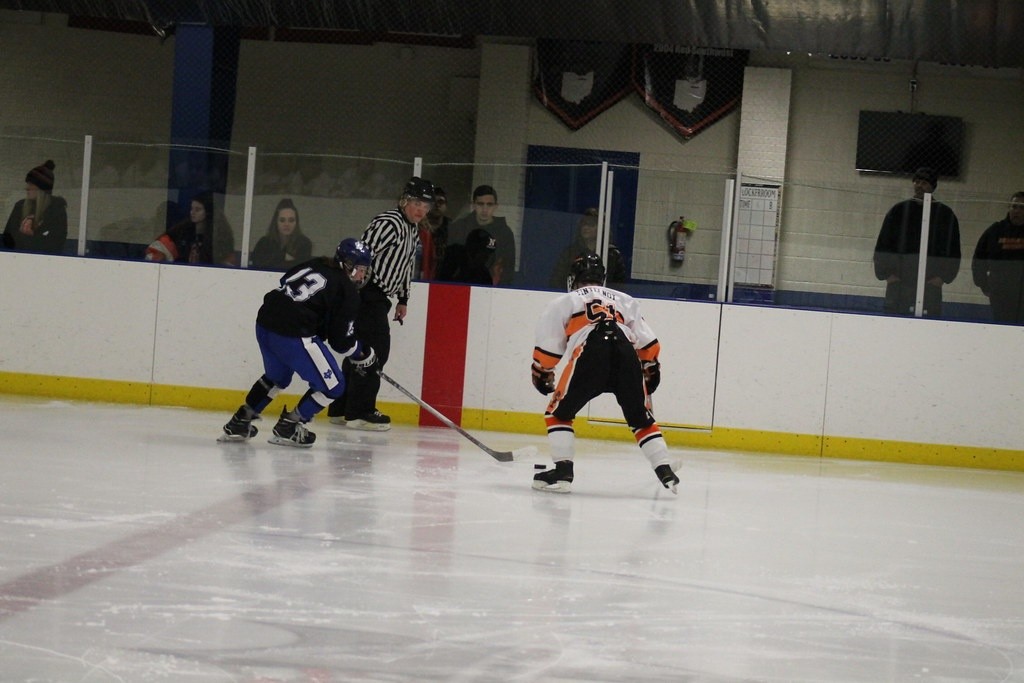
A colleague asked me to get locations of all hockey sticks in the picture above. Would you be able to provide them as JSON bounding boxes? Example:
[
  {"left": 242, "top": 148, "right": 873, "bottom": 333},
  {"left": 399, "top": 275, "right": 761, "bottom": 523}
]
[{"left": 377, "top": 370, "right": 539, "bottom": 462}]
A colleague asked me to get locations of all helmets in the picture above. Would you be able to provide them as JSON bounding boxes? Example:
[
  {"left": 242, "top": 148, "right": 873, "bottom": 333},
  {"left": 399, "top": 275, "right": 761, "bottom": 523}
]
[
  {"left": 568, "top": 251, "right": 607, "bottom": 281},
  {"left": 404, "top": 176, "right": 437, "bottom": 202},
  {"left": 334, "top": 238, "right": 374, "bottom": 272}
]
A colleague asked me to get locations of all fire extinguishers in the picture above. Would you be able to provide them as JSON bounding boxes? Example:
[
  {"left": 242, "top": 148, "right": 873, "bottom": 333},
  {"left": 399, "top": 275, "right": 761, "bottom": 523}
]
[{"left": 667, "top": 215, "right": 689, "bottom": 262}]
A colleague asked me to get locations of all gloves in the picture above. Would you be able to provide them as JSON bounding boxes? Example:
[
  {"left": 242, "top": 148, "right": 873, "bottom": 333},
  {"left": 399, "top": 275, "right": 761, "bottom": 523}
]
[
  {"left": 642, "top": 356, "right": 660, "bottom": 394},
  {"left": 531, "top": 363, "right": 556, "bottom": 396},
  {"left": 350, "top": 346, "right": 382, "bottom": 382}
]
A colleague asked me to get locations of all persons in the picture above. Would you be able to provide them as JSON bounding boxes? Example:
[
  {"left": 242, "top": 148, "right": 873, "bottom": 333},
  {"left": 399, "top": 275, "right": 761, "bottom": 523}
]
[
  {"left": 0, "top": 159, "right": 70, "bottom": 254},
  {"left": 971, "top": 190, "right": 1024, "bottom": 328},
  {"left": 216, "top": 238, "right": 381, "bottom": 448},
  {"left": 416, "top": 185, "right": 517, "bottom": 289},
  {"left": 873, "top": 167, "right": 961, "bottom": 320},
  {"left": 551, "top": 207, "right": 630, "bottom": 293},
  {"left": 250, "top": 197, "right": 311, "bottom": 274},
  {"left": 527, "top": 252, "right": 679, "bottom": 495},
  {"left": 329, "top": 176, "right": 436, "bottom": 432},
  {"left": 146, "top": 195, "right": 236, "bottom": 270}
]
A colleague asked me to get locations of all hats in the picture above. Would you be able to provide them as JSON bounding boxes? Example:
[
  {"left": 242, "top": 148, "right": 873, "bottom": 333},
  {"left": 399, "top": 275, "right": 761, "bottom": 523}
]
[
  {"left": 912, "top": 167, "right": 938, "bottom": 191},
  {"left": 26, "top": 160, "right": 56, "bottom": 194},
  {"left": 149, "top": 235, "right": 179, "bottom": 263}
]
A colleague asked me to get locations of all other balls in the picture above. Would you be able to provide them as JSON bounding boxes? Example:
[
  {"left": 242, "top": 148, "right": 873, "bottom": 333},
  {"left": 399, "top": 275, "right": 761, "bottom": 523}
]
[{"left": 534, "top": 464, "right": 546, "bottom": 469}]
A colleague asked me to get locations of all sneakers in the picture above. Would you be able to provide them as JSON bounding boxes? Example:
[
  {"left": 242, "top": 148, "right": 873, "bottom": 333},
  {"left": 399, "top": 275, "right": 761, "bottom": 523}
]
[
  {"left": 345, "top": 407, "right": 391, "bottom": 431},
  {"left": 532, "top": 460, "right": 575, "bottom": 493},
  {"left": 654, "top": 464, "right": 680, "bottom": 495},
  {"left": 217, "top": 405, "right": 263, "bottom": 443},
  {"left": 268, "top": 404, "right": 316, "bottom": 448},
  {"left": 328, "top": 400, "right": 346, "bottom": 424}
]
